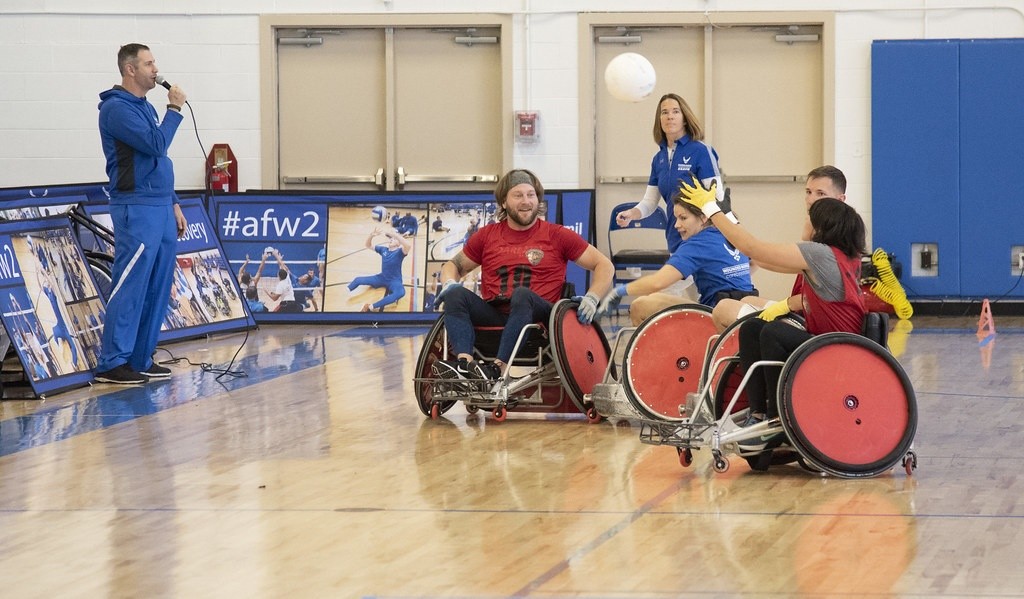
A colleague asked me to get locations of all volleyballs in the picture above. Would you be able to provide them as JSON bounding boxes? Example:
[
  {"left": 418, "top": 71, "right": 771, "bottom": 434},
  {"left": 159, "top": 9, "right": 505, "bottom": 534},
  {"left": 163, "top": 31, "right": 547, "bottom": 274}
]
[
  {"left": 604, "top": 52, "right": 657, "bottom": 102},
  {"left": 371, "top": 205, "right": 388, "bottom": 223},
  {"left": 263, "top": 246, "right": 274, "bottom": 257}
]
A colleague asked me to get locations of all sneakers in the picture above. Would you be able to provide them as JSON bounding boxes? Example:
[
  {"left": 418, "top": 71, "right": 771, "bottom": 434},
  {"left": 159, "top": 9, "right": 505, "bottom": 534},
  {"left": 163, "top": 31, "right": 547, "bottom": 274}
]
[
  {"left": 735, "top": 415, "right": 763, "bottom": 444},
  {"left": 457, "top": 357, "right": 472, "bottom": 385},
  {"left": 93, "top": 362, "right": 148, "bottom": 383},
  {"left": 140, "top": 363, "right": 170, "bottom": 377},
  {"left": 473, "top": 361, "right": 503, "bottom": 390},
  {"left": 738, "top": 432, "right": 786, "bottom": 457}
]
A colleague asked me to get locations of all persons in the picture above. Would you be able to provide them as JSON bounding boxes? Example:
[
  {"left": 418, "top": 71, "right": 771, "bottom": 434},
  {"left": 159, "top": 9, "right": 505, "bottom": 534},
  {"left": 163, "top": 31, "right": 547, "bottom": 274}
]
[
  {"left": 616, "top": 93, "right": 725, "bottom": 298},
  {"left": 164, "top": 252, "right": 235, "bottom": 330},
  {"left": 424, "top": 202, "right": 503, "bottom": 313},
  {"left": 0, "top": 204, "right": 115, "bottom": 380},
  {"left": 431, "top": 167, "right": 614, "bottom": 391},
  {"left": 678, "top": 163, "right": 867, "bottom": 451},
  {"left": 239, "top": 242, "right": 326, "bottom": 312},
  {"left": 596, "top": 189, "right": 759, "bottom": 327},
  {"left": 93, "top": 44, "right": 187, "bottom": 383},
  {"left": 345, "top": 210, "right": 427, "bottom": 312}
]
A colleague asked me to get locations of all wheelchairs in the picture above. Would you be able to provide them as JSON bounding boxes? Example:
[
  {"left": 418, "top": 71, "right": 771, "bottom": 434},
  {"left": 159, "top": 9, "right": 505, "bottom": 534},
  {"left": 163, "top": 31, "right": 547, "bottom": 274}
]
[
  {"left": 582, "top": 302, "right": 719, "bottom": 432},
  {"left": 638, "top": 306, "right": 918, "bottom": 477},
  {"left": 412, "top": 281, "right": 617, "bottom": 421}
]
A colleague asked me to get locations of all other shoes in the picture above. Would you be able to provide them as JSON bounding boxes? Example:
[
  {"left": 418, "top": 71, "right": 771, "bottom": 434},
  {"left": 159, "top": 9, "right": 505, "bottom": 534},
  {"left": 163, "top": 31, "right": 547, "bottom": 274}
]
[{"left": 361, "top": 304, "right": 370, "bottom": 312}]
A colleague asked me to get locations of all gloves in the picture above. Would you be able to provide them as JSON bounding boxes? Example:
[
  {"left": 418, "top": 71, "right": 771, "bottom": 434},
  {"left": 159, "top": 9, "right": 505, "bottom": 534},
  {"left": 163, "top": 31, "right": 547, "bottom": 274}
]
[
  {"left": 433, "top": 279, "right": 461, "bottom": 308},
  {"left": 593, "top": 284, "right": 627, "bottom": 322},
  {"left": 675, "top": 170, "right": 720, "bottom": 218},
  {"left": 758, "top": 297, "right": 789, "bottom": 322},
  {"left": 689, "top": 187, "right": 739, "bottom": 226},
  {"left": 572, "top": 292, "right": 601, "bottom": 325}
]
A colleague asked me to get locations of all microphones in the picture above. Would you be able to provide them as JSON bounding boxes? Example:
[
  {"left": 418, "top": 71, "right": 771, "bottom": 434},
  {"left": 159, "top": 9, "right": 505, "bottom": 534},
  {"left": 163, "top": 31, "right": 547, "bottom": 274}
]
[{"left": 156, "top": 76, "right": 172, "bottom": 90}]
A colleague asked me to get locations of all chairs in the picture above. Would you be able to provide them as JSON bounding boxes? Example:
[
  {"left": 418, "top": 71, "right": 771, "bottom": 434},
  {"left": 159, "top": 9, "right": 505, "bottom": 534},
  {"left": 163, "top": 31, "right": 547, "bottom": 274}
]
[{"left": 605, "top": 201, "right": 671, "bottom": 319}]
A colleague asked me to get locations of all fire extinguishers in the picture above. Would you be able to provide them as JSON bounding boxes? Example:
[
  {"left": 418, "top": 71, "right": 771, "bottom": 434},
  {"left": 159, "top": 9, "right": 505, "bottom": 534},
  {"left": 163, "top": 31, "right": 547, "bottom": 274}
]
[{"left": 209, "top": 161, "right": 233, "bottom": 192}]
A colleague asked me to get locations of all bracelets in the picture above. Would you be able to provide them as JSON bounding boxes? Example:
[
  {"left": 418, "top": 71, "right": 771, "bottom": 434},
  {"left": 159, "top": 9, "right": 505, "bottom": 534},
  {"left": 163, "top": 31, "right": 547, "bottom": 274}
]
[{"left": 167, "top": 104, "right": 181, "bottom": 112}]
